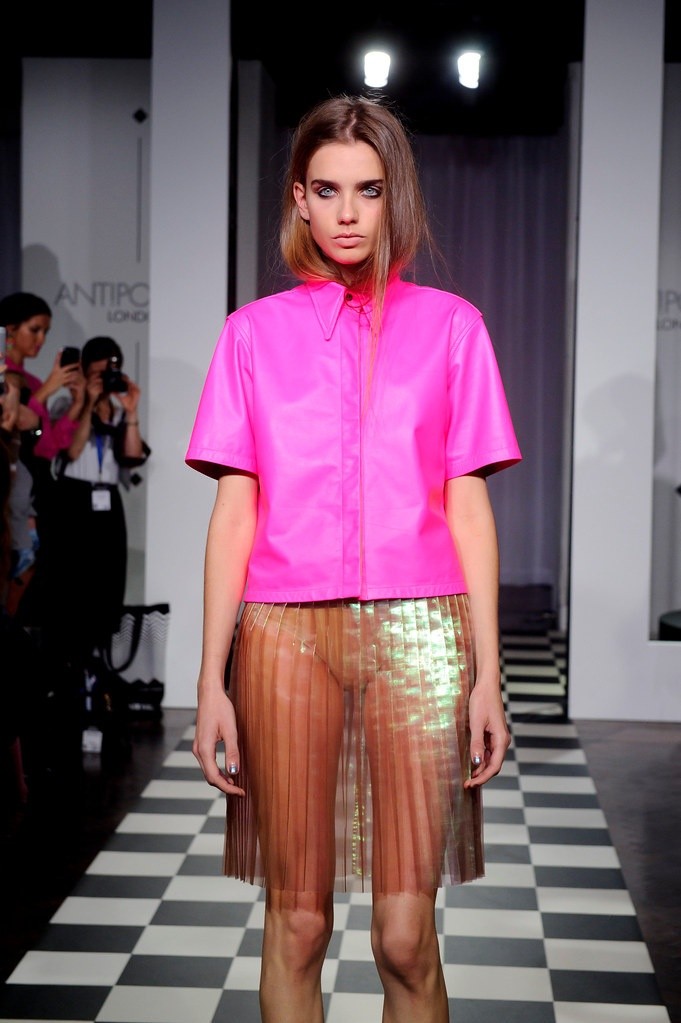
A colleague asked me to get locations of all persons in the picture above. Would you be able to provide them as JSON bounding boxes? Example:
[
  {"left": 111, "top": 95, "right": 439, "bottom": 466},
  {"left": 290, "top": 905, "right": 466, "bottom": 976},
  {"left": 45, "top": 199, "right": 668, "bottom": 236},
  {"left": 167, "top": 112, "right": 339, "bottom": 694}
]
[
  {"left": 183, "top": 97, "right": 523, "bottom": 1023},
  {"left": 0, "top": 293, "right": 151, "bottom": 714}
]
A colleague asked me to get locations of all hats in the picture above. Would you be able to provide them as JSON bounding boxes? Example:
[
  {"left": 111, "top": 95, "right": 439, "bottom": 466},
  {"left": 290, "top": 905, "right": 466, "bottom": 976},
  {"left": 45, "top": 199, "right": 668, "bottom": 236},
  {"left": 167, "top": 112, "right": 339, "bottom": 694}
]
[
  {"left": 81, "top": 337, "right": 123, "bottom": 380},
  {"left": 0, "top": 292, "right": 52, "bottom": 327}
]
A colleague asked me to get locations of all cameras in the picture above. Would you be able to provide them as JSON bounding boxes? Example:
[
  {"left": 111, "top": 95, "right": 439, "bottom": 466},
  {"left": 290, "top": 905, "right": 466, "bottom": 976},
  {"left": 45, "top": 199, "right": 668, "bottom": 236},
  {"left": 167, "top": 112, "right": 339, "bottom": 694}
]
[{"left": 99, "top": 356, "right": 128, "bottom": 393}]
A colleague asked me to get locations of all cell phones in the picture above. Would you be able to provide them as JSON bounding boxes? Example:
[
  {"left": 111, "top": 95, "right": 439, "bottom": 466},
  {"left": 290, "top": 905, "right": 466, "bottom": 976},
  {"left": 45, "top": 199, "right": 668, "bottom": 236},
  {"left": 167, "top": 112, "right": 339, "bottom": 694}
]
[
  {"left": 61, "top": 346, "right": 80, "bottom": 372},
  {"left": 0, "top": 327, "right": 7, "bottom": 383}
]
